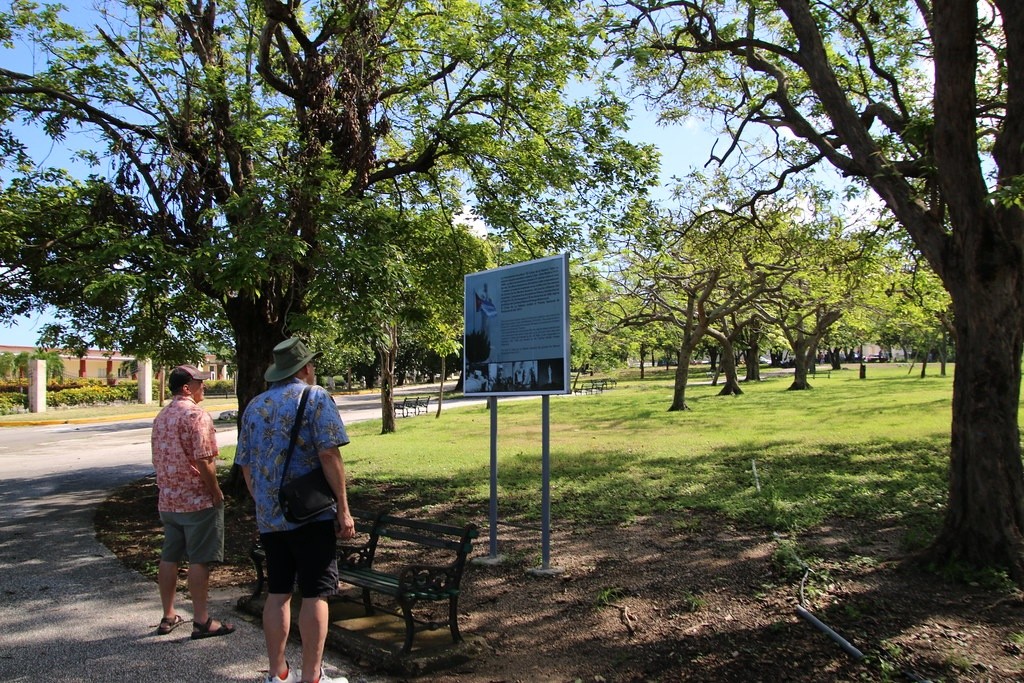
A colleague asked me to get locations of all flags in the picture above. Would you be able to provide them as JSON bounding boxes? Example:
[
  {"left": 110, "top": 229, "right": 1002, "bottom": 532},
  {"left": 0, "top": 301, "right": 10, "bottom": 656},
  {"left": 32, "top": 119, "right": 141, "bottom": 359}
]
[{"left": 474, "top": 289, "right": 498, "bottom": 317}]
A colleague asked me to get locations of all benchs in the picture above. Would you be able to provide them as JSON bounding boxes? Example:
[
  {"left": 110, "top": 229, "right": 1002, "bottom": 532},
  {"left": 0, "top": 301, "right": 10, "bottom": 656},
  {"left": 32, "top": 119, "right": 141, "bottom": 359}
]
[
  {"left": 406, "top": 396, "right": 431, "bottom": 416},
  {"left": 246, "top": 509, "right": 380, "bottom": 609},
  {"left": 394, "top": 397, "right": 419, "bottom": 417},
  {"left": 324, "top": 514, "right": 483, "bottom": 655}
]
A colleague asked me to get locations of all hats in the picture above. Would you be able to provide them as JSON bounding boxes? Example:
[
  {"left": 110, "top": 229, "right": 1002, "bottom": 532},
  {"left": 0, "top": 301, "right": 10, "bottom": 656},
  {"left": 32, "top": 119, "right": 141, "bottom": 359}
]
[
  {"left": 264, "top": 337, "right": 324, "bottom": 381},
  {"left": 168, "top": 364, "right": 210, "bottom": 387}
]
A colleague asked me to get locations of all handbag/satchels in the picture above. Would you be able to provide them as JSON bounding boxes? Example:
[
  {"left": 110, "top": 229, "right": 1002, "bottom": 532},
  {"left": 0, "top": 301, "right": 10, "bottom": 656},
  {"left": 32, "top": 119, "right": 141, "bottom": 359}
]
[{"left": 278, "top": 385, "right": 336, "bottom": 525}]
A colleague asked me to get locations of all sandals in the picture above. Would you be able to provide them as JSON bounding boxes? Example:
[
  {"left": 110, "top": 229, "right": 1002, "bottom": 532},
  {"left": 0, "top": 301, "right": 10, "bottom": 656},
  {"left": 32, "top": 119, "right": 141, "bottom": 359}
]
[
  {"left": 159, "top": 615, "right": 184, "bottom": 632},
  {"left": 191, "top": 617, "right": 234, "bottom": 639}
]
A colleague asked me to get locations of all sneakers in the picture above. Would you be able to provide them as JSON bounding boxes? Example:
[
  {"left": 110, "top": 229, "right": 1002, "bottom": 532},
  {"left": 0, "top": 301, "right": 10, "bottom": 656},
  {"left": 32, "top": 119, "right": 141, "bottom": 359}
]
[
  {"left": 319, "top": 667, "right": 349, "bottom": 683},
  {"left": 265, "top": 661, "right": 302, "bottom": 683}
]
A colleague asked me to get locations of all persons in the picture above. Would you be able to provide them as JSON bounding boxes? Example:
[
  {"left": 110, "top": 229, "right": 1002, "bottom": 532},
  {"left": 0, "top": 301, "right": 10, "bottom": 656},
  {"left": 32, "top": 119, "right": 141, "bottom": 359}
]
[
  {"left": 466, "top": 360, "right": 563, "bottom": 393},
  {"left": 233, "top": 336, "right": 355, "bottom": 683},
  {"left": 155, "top": 364, "right": 235, "bottom": 640}
]
